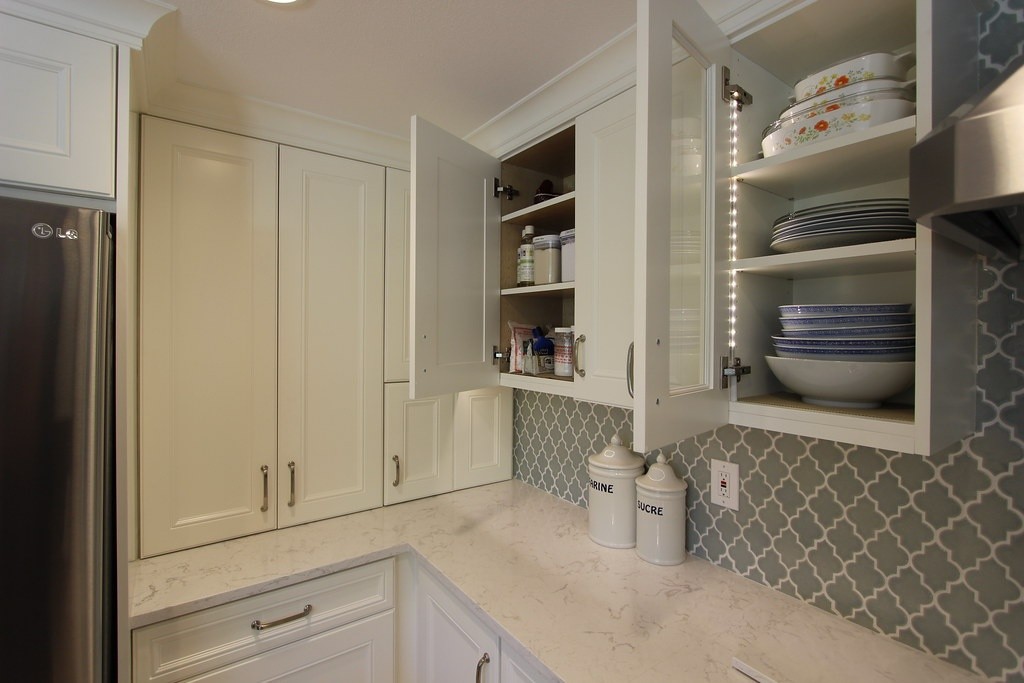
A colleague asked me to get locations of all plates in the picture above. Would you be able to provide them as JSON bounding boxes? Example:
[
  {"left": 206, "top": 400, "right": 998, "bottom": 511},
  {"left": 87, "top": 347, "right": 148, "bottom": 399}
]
[{"left": 767, "top": 196, "right": 915, "bottom": 253}]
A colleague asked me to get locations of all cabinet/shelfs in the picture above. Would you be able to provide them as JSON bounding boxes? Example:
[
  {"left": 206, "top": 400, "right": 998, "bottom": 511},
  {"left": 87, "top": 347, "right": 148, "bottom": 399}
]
[
  {"left": 409, "top": 54, "right": 707, "bottom": 413},
  {"left": 382, "top": 167, "right": 515, "bottom": 512},
  {"left": 139, "top": 113, "right": 383, "bottom": 562},
  {"left": 128, "top": 559, "right": 398, "bottom": 683},
  {"left": 630, "top": 0, "right": 977, "bottom": 458},
  {"left": 407, "top": 553, "right": 542, "bottom": 683}
]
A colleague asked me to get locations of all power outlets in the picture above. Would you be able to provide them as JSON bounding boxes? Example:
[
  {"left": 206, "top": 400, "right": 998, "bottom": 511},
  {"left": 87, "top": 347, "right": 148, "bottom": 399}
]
[{"left": 709, "top": 459, "right": 740, "bottom": 513}]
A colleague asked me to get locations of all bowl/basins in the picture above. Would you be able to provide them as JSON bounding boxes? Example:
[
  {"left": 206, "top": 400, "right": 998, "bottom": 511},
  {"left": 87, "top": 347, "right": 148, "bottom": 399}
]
[
  {"left": 761, "top": 50, "right": 917, "bottom": 159},
  {"left": 763, "top": 300, "right": 915, "bottom": 413}
]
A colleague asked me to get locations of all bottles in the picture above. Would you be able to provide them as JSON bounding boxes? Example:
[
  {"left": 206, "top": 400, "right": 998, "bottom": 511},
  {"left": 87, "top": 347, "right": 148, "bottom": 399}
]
[{"left": 515, "top": 225, "right": 534, "bottom": 286}]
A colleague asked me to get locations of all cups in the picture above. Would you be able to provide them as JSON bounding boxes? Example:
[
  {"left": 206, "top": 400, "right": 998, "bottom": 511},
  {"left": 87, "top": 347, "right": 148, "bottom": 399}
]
[
  {"left": 587, "top": 435, "right": 687, "bottom": 566},
  {"left": 553, "top": 327, "right": 574, "bottom": 377},
  {"left": 558, "top": 228, "right": 576, "bottom": 282},
  {"left": 532, "top": 235, "right": 561, "bottom": 285}
]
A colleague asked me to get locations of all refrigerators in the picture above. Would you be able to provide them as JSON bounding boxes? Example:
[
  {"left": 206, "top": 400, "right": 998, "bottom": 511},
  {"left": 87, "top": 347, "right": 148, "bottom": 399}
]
[{"left": 3, "top": 193, "right": 116, "bottom": 683}]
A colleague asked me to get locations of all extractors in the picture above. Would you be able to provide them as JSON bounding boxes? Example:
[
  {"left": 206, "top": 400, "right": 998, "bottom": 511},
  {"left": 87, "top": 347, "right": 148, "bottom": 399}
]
[{"left": 909, "top": 50, "right": 1023, "bottom": 267}]
